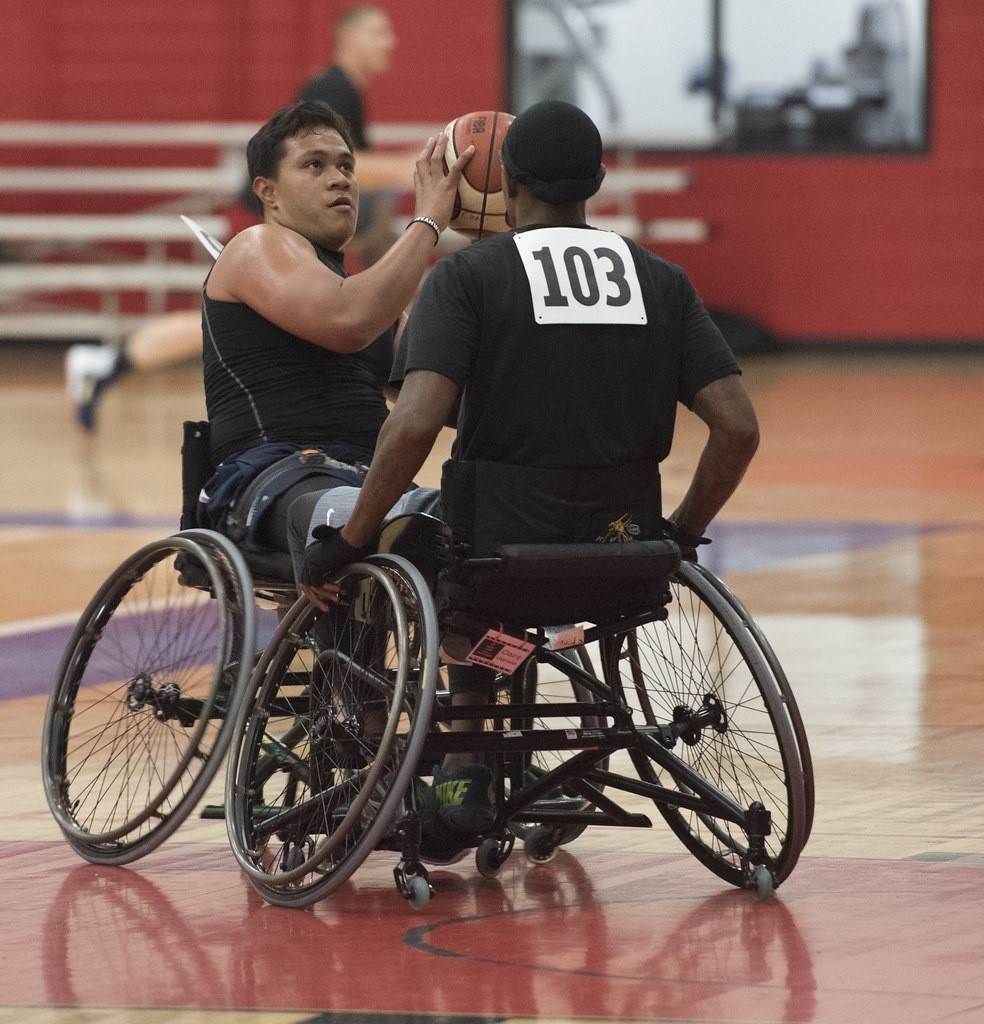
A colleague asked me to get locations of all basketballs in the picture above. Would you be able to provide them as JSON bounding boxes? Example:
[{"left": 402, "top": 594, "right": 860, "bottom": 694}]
[{"left": 432, "top": 108, "right": 528, "bottom": 235}]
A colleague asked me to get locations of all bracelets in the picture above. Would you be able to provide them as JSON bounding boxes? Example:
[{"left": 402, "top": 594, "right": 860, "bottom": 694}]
[{"left": 404, "top": 217, "right": 441, "bottom": 247}]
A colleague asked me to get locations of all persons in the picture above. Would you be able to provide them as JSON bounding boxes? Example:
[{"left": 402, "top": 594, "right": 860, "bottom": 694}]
[
  {"left": 65, "top": 5, "right": 396, "bottom": 433},
  {"left": 198, "top": 98, "right": 501, "bottom": 837},
  {"left": 298, "top": 95, "right": 760, "bottom": 866}
]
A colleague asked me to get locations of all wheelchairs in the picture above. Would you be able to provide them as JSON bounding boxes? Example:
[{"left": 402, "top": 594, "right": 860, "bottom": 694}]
[{"left": 39, "top": 423, "right": 814, "bottom": 908}]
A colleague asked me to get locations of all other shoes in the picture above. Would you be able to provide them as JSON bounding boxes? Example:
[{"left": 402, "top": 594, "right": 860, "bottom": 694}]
[
  {"left": 65, "top": 341, "right": 119, "bottom": 441},
  {"left": 428, "top": 762, "right": 495, "bottom": 831}
]
